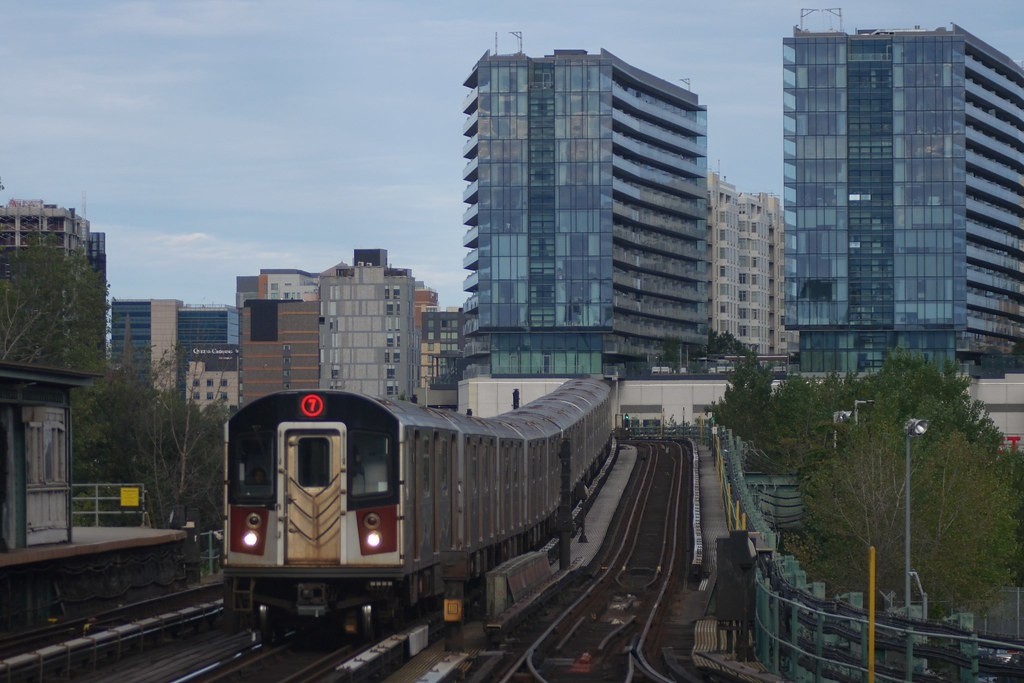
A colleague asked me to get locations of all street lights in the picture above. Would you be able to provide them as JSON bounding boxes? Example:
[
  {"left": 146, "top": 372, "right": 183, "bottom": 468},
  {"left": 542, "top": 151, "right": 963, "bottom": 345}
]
[
  {"left": 903, "top": 418, "right": 929, "bottom": 607},
  {"left": 833, "top": 411, "right": 852, "bottom": 450},
  {"left": 854, "top": 399, "right": 876, "bottom": 426}
]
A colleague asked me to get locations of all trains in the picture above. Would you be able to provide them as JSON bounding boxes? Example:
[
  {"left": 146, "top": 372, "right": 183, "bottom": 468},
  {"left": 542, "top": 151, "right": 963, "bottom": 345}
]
[{"left": 221, "top": 378, "right": 615, "bottom": 647}]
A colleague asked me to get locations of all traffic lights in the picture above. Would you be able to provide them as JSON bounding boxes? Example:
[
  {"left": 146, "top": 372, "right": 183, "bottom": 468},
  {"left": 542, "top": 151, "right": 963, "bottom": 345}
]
[{"left": 625, "top": 415, "right": 628, "bottom": 420}]
